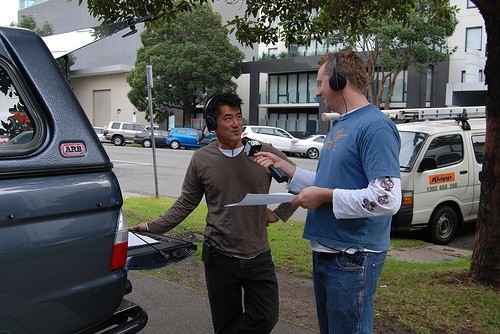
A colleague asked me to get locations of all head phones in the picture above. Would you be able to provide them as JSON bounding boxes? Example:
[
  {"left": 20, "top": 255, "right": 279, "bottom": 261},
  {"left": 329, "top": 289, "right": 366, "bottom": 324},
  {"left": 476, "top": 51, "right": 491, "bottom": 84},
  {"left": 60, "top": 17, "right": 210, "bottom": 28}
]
[
  {"left": 328, "top": 52, "right": 347, "bottom": 91},
  {"left": 204, "top": 94, "right": 219, "bottom": 134}
]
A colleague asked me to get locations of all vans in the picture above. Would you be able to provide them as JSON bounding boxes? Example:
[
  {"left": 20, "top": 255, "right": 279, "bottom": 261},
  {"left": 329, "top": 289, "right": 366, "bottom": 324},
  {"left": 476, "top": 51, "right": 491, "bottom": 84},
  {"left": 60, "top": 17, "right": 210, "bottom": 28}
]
[
  {"left": 239, "top": 125, "right": 302, "bottom": 154},
  {"left": 390, "top": 114, "right": 487, "bottom": 243},
  {"left": 0, "top": 27, "right": 199, "bottom": 334}
]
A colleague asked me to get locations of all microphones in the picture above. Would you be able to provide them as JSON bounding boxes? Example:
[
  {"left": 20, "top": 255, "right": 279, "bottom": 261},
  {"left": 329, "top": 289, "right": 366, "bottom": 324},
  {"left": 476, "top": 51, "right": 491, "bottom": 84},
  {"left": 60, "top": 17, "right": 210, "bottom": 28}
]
[{"left": 241, "top": 136, "right": 288, "bottom": 184}]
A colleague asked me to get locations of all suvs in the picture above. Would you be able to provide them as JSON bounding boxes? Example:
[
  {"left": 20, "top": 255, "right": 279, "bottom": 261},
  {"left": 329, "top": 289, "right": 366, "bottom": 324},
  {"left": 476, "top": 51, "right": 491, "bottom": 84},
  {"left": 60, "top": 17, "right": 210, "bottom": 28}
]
[
  {"left": 197, "top": 124, "right": 218, "bottom": 148},
  {"left": 102, "top": 120, "right": 147, "bottom": 146}
]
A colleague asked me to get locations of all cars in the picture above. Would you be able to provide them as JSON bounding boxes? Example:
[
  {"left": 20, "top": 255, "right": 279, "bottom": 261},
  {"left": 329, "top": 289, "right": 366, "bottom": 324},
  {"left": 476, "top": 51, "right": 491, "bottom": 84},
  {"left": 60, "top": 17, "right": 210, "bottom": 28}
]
[
  {"left": 165, "top": 128, "right": 206, "bottom": 149},
  {"left": 290, "top": 134, "right": 327, "bottom": 159},
  {"left": 133, "top": 128, "right": 171, "bottom": 148}
]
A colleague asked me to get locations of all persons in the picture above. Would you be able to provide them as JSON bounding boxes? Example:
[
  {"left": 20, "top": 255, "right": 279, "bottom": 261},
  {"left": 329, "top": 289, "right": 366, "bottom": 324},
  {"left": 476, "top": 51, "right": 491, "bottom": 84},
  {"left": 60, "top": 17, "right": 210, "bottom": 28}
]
[
  {"left": 126, "top": 90, "right": 298, "bottom": 334},
  {"left": 254, "top": 49, "right": 402, "bottom": 334}
]
